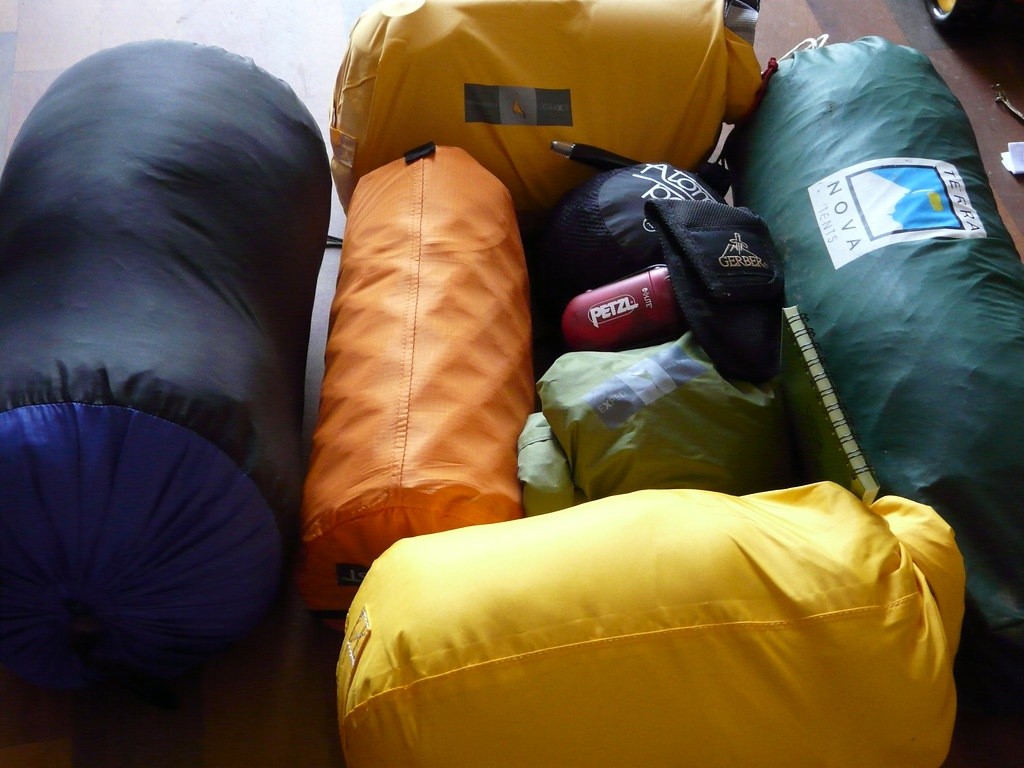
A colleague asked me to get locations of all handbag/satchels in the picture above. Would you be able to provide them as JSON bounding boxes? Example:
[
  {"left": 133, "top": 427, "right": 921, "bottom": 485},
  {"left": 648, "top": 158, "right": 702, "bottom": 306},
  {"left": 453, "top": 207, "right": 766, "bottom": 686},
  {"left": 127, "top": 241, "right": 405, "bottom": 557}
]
[{"left": 2, "top": 2, "right": 1022, "bottom": 767}]
